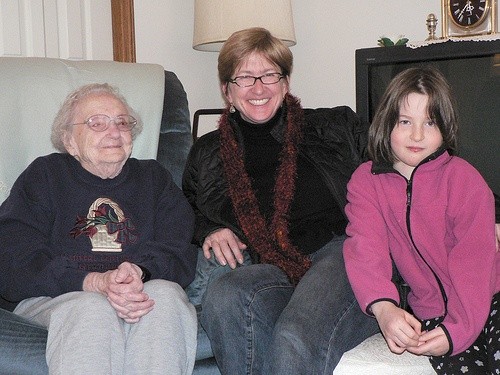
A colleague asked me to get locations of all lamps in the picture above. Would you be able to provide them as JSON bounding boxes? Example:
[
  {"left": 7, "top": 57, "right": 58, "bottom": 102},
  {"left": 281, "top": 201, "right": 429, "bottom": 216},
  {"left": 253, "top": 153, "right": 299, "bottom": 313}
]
[{"left": 192, "top": 1, "right": 297, "bottom": 53}]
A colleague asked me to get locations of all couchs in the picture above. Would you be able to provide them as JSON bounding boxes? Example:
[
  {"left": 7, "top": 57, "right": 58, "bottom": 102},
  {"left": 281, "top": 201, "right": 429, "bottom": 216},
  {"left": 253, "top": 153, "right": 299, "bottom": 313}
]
[{"left": 0, "top": 56, "right": 222, "bottom": 375}]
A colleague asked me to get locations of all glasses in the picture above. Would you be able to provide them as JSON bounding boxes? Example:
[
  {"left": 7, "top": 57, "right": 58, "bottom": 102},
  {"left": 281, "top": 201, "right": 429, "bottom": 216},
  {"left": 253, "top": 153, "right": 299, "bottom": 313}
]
[
  {"left": 229, "top": 73, "right": 285, "bottom": 87},
  {"left": 72, "top": 114, "right": 138, "bottom": 132}
]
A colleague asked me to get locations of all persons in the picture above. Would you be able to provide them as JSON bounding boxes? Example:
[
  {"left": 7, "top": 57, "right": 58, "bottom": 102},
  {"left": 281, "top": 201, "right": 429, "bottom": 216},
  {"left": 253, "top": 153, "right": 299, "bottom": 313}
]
[
  {"left": 342, "top": 67, "right": 500, "bottom": 375},
  {"left": 1, "top": 83, "right": 197, "bottom": 375},
  {"left": 182, "top": 28, "right": 413, "bottom": 375}
]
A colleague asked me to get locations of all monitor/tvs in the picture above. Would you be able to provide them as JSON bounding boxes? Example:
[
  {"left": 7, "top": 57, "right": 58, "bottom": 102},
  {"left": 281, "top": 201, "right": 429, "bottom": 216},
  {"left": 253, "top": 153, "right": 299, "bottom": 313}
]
[{"left": 353, "top": 39, "right": 500, "bottom": 223}]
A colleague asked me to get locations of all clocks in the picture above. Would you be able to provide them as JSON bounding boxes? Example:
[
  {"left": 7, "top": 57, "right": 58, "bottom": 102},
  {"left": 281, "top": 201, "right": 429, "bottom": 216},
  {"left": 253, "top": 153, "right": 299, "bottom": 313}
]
[{"left": 441, "top": 0, "right": 497, "bottom": 37}]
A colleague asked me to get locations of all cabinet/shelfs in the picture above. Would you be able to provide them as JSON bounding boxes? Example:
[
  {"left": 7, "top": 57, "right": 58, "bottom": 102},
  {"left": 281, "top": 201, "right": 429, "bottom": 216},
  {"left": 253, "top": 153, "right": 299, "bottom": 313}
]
[{"left": 354, "top": 39, "right": 500, "bottom": 226}]
